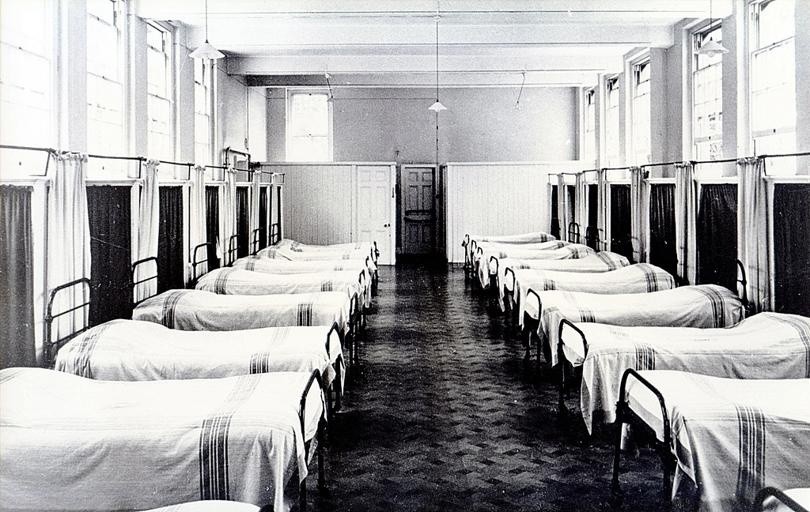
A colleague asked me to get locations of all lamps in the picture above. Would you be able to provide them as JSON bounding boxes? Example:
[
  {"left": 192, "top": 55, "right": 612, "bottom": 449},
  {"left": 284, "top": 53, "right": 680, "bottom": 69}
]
[
  {"left": 189, "top": 0, "right": 224, "bottom": 60},
  {"left": 428, "top": 16, "right": 447, "bottom": 112},
  {"left": 699, "top": 0, "right": 729, "bottom": 57}
]
